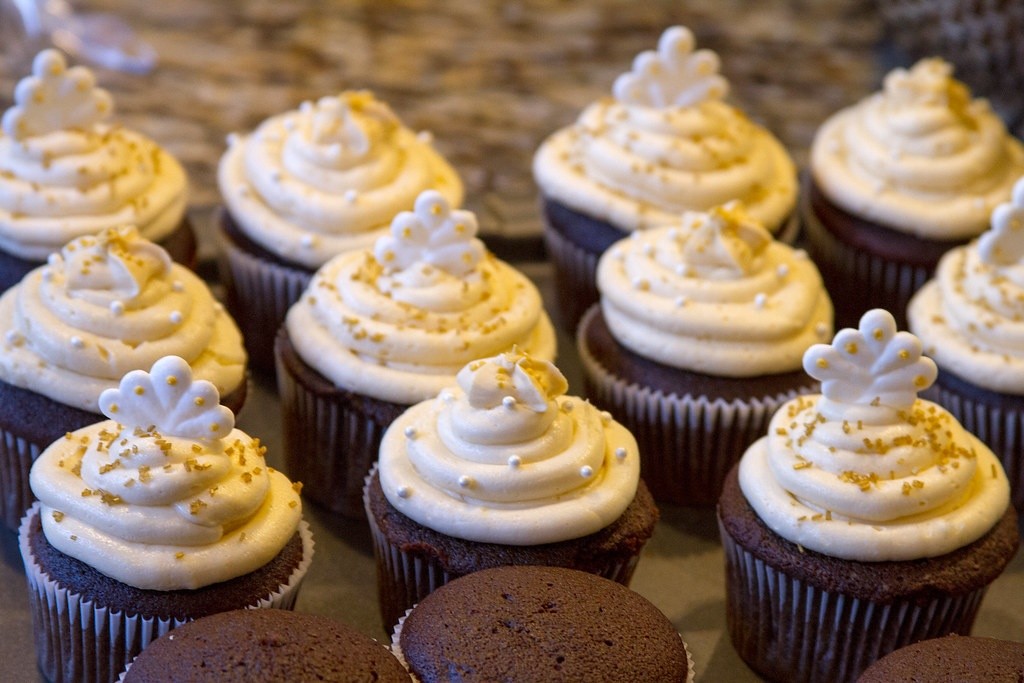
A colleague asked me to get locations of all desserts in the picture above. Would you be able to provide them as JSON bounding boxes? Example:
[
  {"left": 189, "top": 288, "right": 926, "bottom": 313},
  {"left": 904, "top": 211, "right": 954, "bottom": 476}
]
[{"left": 0, "top": 27, "right": 1024, "bottom": 683}]
